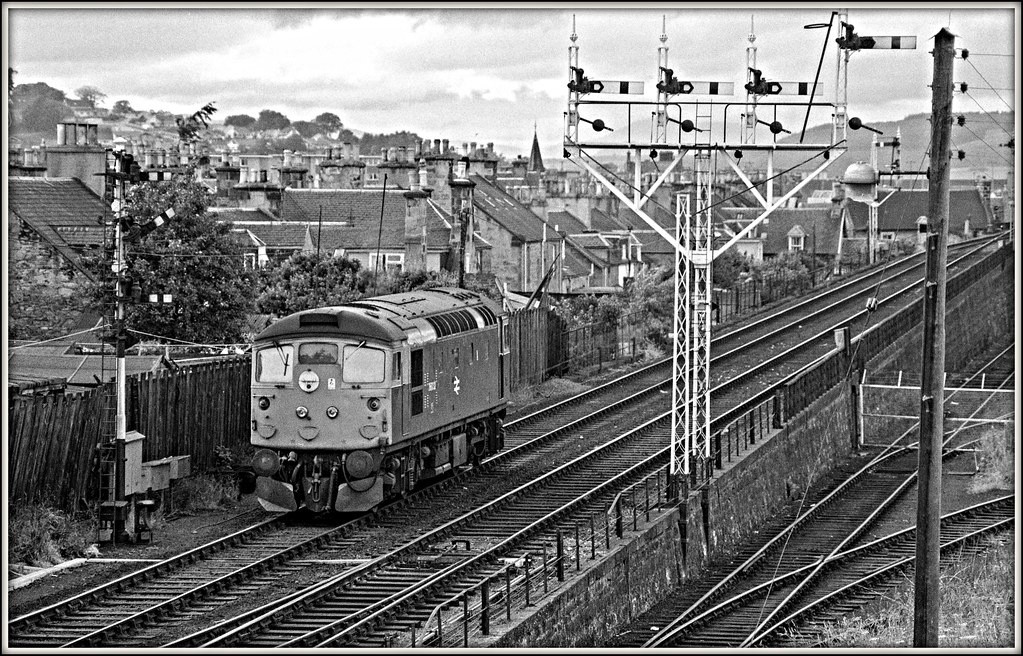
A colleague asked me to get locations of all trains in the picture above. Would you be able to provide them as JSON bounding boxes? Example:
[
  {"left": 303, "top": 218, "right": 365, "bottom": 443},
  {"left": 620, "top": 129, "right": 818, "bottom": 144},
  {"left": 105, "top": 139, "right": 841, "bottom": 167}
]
[{"left": 231, "top": 287, "right": 512, "bottom": 520}]
